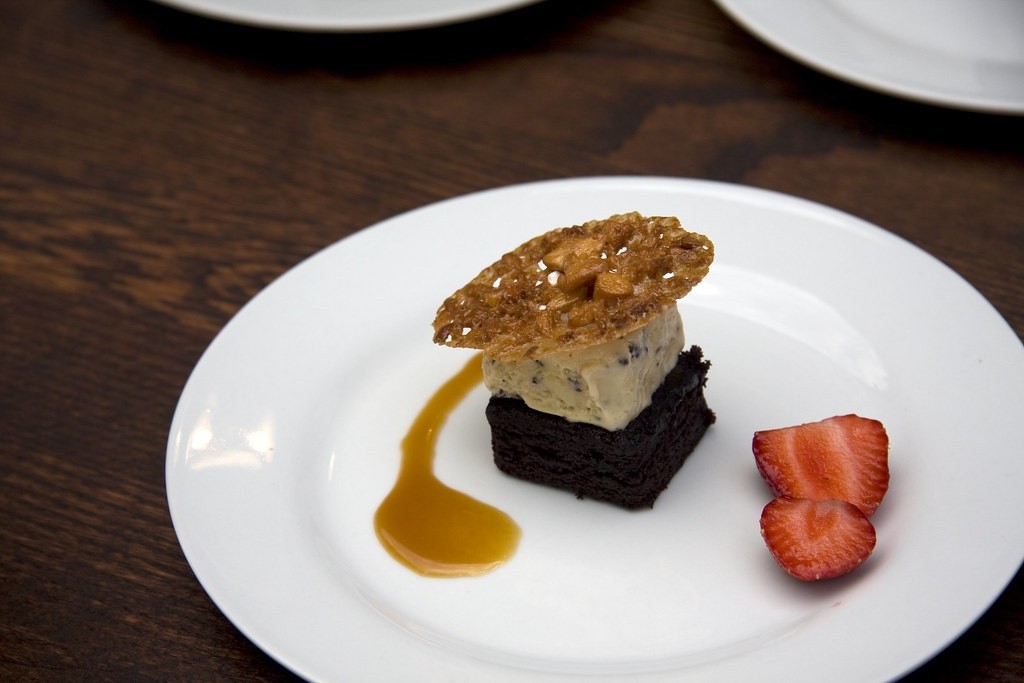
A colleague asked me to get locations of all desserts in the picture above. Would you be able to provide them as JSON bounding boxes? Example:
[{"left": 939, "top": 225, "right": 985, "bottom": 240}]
[{"left": 432, "top": 211, "right": 716, "bottom": 510}]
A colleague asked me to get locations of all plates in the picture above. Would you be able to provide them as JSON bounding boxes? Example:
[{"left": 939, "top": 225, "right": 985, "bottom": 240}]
[
  {"left": 714, "top": 0, "right": 1023, "bottom": 111},
  {"left": 162, "top": 176, "right": 1024, "bottom": 683},
  {"left": 167, "top": 1, "right": 530, "bottom": 30}
]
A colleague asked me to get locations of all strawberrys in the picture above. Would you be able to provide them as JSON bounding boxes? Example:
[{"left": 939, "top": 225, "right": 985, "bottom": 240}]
[
  {"left": 760, "top": 494, "right": 877, "bottom": 582},
  {"left": 752, "top": 414, "right": 891, "bottom": 519}
]
